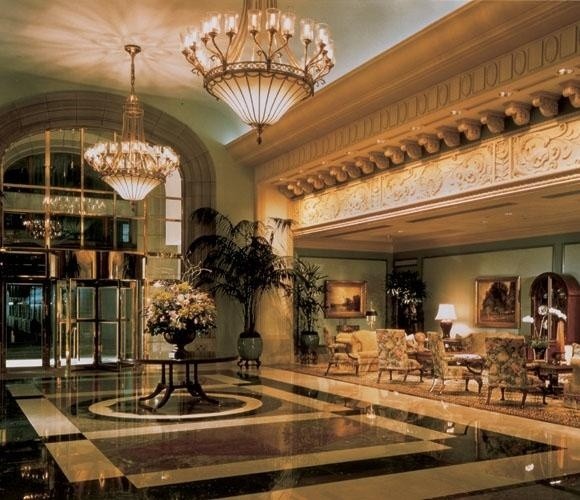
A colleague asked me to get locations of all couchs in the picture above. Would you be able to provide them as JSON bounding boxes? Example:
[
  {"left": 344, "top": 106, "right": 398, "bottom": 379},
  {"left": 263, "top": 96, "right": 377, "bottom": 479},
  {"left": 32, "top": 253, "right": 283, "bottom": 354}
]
[{"left": 324, "top": 328, "right": 580, "bottom": 409}]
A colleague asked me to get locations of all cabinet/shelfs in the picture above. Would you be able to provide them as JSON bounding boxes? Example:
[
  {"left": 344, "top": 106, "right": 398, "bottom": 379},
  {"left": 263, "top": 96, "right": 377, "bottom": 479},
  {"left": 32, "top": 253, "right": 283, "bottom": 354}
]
[{"left": 528, "top": 272, "right": 580, "bottom": 345}]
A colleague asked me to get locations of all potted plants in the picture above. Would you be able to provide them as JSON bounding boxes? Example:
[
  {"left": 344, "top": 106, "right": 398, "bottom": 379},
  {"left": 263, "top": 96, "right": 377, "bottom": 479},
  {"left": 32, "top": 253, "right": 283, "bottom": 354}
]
[
  {"left": 184, "top": 206, "right": 311, "bottom": 361},
  {"left": 282, "top": 258, "right": 331, "bottom": 360}
]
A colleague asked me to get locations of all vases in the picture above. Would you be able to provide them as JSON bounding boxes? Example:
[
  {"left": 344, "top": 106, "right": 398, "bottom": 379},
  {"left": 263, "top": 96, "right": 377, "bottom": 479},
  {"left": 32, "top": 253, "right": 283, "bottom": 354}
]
[{"left": 163, "top": 330, "right": 196, "bottom": 359}]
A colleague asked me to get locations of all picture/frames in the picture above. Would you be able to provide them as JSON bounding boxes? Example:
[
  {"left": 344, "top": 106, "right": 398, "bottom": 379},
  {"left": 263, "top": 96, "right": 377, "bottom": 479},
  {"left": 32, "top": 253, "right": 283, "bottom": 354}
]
[
  {"left": 324, "top": 280, "right": 368, "bottom": 319},
  {"left": 473, "top": 273, "right": 521, "bottom": 329}
]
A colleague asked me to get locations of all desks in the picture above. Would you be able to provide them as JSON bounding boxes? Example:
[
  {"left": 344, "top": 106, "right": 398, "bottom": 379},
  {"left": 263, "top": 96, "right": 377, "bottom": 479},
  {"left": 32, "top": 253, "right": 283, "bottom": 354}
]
[{"left": 116, "top": 351, "right": 239, "bottom": 415}]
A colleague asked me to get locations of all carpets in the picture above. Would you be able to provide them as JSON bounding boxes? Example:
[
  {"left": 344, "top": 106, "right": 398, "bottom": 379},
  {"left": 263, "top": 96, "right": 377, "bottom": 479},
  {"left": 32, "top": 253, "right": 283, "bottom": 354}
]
[{"left": 279, "top": 367, "right": 580, "bottom": 428}]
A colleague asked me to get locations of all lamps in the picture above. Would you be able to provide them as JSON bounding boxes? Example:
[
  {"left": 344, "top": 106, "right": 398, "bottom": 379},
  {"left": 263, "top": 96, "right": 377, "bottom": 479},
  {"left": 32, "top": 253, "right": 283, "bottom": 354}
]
[
  {"left": 181, "top": 0, "right": 337, "bottom": 145},
  {"left": 84, "top": 44, "right": 181, "bottom": 213},
  {"left": 435, "top": 303, "right": 458, "bottom": 339},
  {"left": 364, "top": 309, "right": 379, "bottom": 328}
]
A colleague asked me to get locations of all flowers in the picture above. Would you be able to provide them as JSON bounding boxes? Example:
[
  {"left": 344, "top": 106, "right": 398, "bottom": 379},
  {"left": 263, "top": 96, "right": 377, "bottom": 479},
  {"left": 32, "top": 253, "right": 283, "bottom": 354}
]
[
  {"left": 143, "top": 281, "right": 219, "bottom": 338},
  {"left": 522, "top": 304, "right": 567, "bottom": 348}
]
